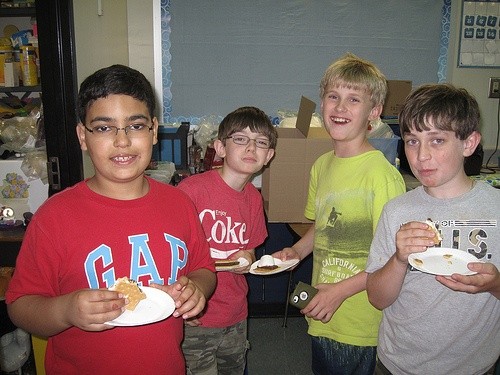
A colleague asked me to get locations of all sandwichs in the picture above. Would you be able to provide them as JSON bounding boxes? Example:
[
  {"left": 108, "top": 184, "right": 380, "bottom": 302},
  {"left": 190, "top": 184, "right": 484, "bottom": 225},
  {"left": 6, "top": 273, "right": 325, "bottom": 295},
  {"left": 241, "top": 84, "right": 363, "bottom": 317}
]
[{"left": 214, "top": 260, "right": 239, "bottom": 269}]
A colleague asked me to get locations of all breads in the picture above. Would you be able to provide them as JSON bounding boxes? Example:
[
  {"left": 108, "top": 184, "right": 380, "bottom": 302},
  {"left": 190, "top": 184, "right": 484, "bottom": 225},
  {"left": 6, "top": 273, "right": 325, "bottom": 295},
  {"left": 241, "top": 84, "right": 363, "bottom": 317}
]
[
  {"left": 108, "top": 276, "right": 147, "bottom": 311},
  {"left": 421, "top": 219, "right": 442, "bottom": 245}
]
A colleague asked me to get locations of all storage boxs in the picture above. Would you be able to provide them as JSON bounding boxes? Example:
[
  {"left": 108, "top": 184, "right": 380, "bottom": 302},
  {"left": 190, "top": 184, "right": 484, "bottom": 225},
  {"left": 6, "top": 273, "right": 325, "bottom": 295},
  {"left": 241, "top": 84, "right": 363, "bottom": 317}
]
[
  {"left": 366, "top": 134, "right": 401, "bottom": 167},
  {"left": 260, "top": 95, "right": 335, "bottom": 223},
  {"left": 382, "top": 80, "right": 412, "bottom": 116}
]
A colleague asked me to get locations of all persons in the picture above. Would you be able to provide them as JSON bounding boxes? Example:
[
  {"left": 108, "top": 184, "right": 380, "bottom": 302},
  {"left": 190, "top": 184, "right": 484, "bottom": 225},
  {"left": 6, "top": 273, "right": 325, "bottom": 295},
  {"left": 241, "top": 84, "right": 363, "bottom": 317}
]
[
  {"left": 4, "top": 65, "right": 218, "bottom": 375},
  {"left": 365, "top": 81, "right": 500, "bottom": 375},
  {"left": 176, "top": 107, "right": 277, "bottom": 375},
  {"left": 268, "top": 54, "right": 407, "bottom": 375}
]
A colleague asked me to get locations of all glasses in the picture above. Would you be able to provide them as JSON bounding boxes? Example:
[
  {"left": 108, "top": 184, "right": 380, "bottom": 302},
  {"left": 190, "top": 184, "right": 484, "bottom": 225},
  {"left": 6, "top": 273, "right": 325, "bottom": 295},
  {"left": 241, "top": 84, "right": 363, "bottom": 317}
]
[
  {"left": 85, "top": 124, "right": 154, "bottom": 139},
  {"left": 225, "top": 135, "right": 271, "bottom": 149}
]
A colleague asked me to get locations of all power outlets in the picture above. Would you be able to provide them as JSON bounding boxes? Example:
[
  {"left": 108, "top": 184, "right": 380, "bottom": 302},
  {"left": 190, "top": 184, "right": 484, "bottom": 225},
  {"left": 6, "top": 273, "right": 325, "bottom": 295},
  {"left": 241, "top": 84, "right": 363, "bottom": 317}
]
[{"left": 487, "top": 77, "right": 500, "bottom": 98}]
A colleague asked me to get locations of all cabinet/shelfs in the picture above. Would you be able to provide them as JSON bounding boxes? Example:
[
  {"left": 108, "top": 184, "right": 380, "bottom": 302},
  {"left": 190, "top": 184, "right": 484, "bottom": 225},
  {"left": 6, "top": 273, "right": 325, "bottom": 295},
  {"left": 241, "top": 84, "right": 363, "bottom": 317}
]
[{"left": 0, "top": 0, "right": 69, "bottom": 267}]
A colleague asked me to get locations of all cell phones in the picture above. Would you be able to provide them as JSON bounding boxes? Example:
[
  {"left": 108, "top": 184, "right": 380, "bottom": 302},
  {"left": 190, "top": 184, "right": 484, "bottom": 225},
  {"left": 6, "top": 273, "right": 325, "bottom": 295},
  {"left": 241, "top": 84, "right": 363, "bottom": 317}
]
[{"left": 290, "top": 282, "right": 319, "bottom": 309}]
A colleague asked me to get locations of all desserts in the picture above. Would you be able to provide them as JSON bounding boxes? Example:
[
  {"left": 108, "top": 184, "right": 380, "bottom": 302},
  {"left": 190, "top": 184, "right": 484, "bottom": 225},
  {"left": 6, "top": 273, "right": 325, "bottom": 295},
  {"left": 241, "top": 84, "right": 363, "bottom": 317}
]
[{"left": 254, "top": 255, "right": 281, "bottom": 273}]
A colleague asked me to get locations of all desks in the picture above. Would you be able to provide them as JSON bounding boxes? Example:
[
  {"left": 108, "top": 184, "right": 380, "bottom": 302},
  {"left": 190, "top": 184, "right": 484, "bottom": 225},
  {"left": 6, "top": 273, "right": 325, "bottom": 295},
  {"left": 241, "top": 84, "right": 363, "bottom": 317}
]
[{"left": 282, "top": 223, "right": 313, "bottom": 330}]
